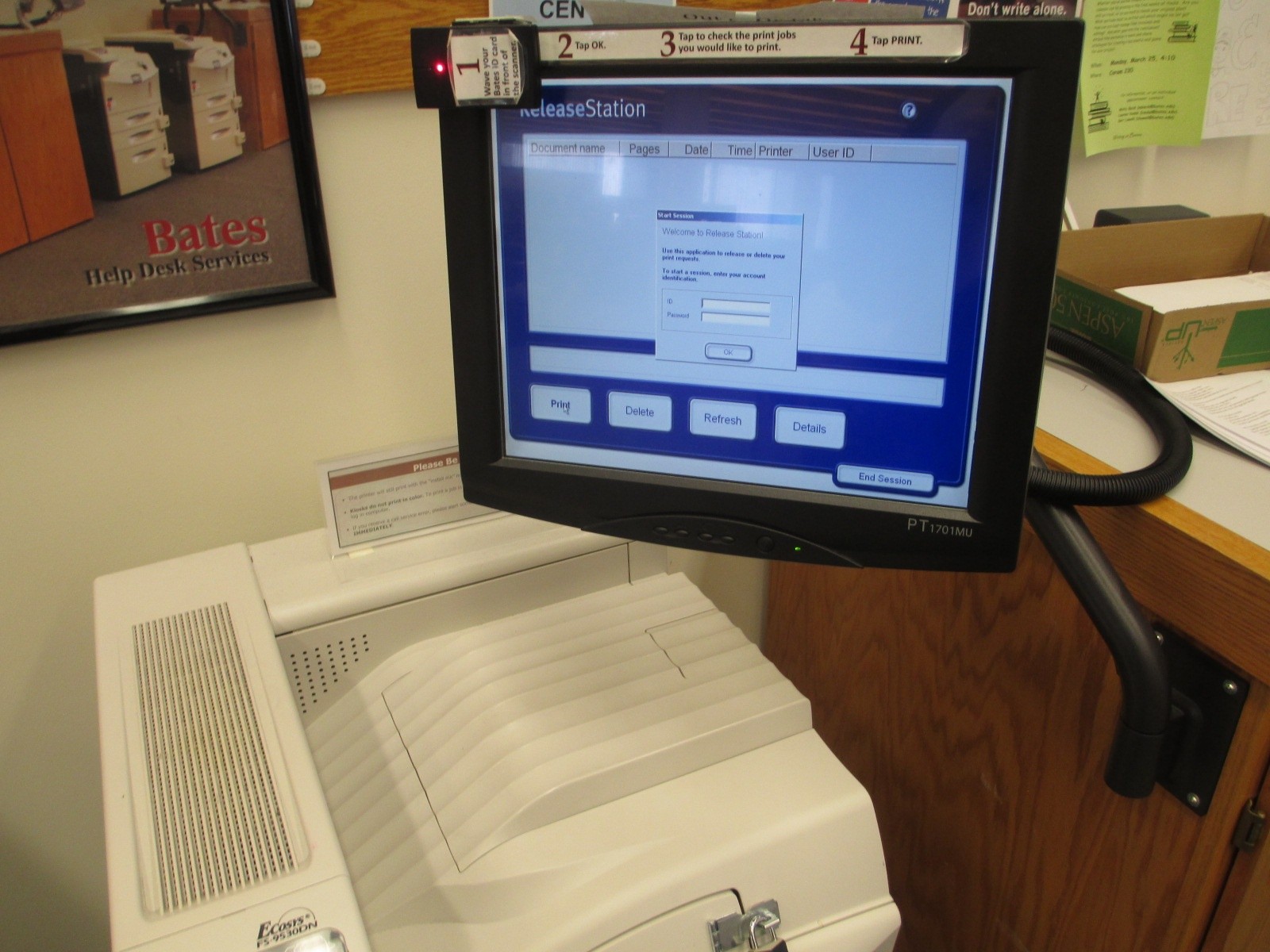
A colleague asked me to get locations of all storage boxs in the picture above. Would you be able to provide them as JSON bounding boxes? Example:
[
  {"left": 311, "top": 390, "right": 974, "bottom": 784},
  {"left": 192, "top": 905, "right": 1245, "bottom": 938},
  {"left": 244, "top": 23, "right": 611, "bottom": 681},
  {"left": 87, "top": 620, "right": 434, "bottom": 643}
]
[{"left": 1044, "top": 211, "right": 1270, "bottom": 385}]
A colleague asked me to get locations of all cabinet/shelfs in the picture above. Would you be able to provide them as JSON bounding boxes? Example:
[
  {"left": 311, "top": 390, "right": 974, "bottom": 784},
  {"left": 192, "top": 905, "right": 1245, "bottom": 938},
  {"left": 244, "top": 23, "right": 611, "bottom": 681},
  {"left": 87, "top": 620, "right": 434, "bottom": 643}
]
[{"left": 762, "top": 359, "right": 1270, "bottom": 952}]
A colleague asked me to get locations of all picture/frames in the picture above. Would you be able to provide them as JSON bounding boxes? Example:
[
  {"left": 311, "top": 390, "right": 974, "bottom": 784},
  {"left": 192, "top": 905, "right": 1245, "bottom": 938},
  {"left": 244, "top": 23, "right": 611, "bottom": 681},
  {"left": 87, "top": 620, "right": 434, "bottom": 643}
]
[{"left": 0, "top": 0, "right": 337, "bottom": 351}]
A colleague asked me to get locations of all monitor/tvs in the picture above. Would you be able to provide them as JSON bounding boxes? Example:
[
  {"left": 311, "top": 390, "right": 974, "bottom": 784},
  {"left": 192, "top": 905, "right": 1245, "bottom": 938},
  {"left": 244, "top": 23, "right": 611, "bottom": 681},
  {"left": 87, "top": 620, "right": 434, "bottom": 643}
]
[{"left": 440, "top": 15, "right": 1085, "bottom": 570}]
